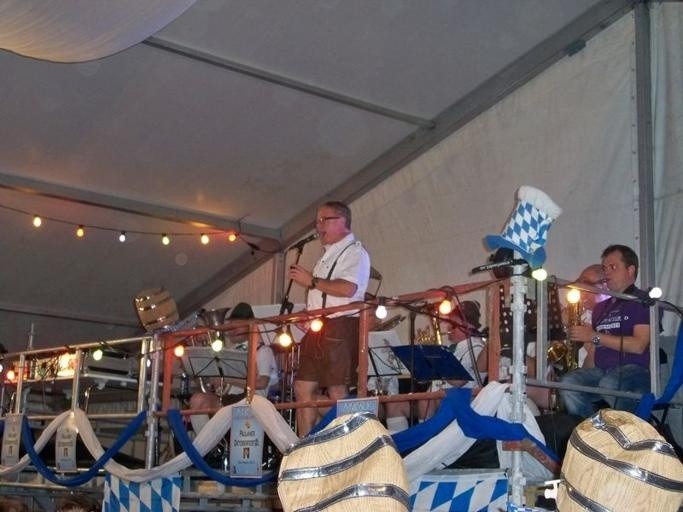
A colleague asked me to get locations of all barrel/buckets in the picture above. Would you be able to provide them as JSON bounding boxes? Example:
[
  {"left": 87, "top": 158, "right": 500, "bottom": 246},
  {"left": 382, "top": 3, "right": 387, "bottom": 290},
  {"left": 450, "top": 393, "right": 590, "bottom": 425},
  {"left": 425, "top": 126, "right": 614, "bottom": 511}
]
[{"left": 133, "top": 287, "right": 179, "bottom": 332}]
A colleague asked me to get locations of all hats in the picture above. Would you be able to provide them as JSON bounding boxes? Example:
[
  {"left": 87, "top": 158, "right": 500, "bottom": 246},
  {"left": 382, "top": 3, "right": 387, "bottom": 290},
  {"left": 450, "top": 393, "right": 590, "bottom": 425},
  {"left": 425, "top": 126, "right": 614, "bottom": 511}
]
[
  {"left": 225, "top": 303, "right": 254, "bottom": 320},
  {"left": 446, "top": 300, "right": 481, "bottom": 329}
]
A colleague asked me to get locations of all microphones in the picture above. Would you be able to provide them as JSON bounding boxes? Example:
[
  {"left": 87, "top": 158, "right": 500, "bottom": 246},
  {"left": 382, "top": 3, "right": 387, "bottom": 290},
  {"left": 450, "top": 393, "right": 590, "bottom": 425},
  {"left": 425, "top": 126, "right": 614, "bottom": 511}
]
[{"left": 285, "top": 230, "right": 321, "bottom": 254}]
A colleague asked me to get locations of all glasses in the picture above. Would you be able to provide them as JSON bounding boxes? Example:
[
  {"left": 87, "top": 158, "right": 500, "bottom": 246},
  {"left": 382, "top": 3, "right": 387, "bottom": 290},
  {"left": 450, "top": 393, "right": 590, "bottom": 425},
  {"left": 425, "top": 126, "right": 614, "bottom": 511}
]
[{"left": 316, "top": 216, "right": 340, "bottom": 224}]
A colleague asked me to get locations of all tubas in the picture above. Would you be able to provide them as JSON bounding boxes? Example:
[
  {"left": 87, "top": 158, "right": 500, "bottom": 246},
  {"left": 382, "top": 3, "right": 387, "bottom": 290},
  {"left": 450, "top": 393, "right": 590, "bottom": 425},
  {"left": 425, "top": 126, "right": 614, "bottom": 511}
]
[{"left": 197, "top": 307, "right": 232, "bottom": 396}]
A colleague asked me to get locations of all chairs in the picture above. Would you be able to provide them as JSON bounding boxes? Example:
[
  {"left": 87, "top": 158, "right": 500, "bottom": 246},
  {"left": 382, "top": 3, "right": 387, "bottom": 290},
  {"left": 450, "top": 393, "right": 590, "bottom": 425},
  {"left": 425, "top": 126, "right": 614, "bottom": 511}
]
[
  {"left": 224, "top": 369, "right": 283, "bottom": 472},
  {"left": 592, "top": 346, "right": 668, "bottom": 439}
]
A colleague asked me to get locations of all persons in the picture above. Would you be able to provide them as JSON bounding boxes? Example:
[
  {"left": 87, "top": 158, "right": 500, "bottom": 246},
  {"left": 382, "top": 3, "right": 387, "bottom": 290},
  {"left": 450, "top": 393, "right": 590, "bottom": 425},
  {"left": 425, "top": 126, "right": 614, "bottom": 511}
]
[
  {"left": 191, "top": 302, "right": 281, "bottom": 434},
  {"left": 287, "top": 201, "right": 372, "bottom": 438},
  {"left": 384, "top": 300, "right": 488, "bottom": 431},
  {"left": 528, "top": 244, "right": 651, "bottom": 418}
]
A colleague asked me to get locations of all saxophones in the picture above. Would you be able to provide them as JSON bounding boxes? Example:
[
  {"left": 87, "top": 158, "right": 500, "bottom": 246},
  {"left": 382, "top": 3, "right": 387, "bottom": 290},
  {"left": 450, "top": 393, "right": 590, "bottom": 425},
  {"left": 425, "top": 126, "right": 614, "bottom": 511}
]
[
  {"left": 547, "top": 278, "right": 606, "bottom": 376},
  {"left": 418, "top": 319, "right": 452, "bottom": 346}
]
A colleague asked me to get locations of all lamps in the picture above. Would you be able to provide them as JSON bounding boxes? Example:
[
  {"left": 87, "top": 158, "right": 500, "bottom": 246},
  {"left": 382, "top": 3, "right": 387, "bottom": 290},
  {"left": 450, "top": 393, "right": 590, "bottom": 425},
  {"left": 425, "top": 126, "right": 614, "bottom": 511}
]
[{"left": 32, "top": 214, "right": 238, "bottom": 246}]
